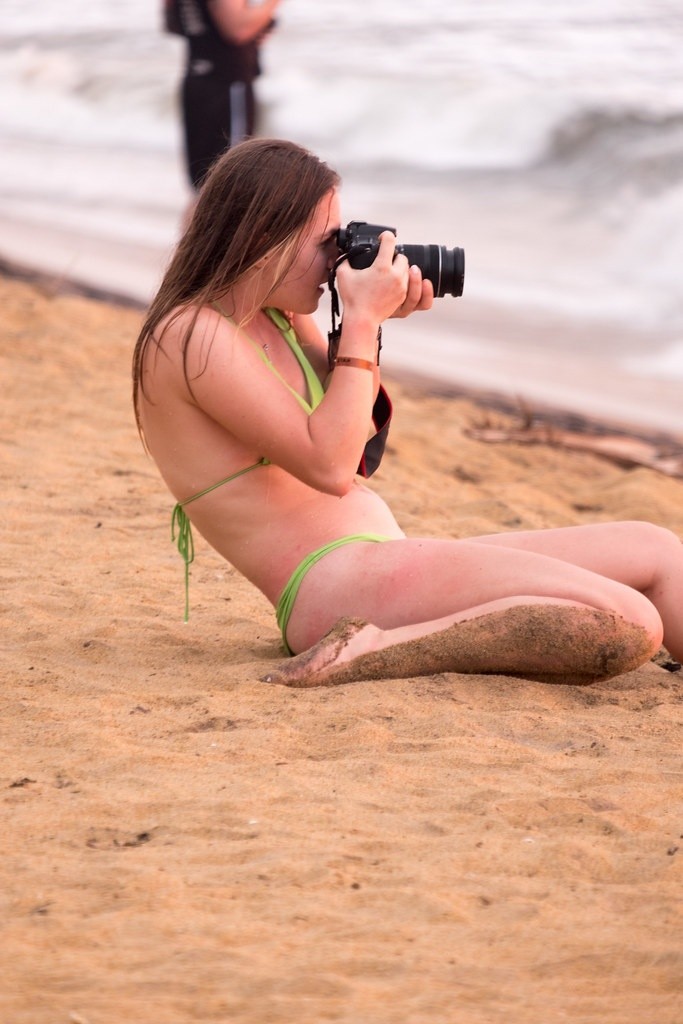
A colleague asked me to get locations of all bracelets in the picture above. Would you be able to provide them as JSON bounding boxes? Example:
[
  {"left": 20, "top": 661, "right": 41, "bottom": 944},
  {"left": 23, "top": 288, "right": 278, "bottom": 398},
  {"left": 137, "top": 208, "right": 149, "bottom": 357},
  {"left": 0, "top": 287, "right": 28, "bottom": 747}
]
[{"left": 335, "top": 356, "right": 374, "bottom": 371}]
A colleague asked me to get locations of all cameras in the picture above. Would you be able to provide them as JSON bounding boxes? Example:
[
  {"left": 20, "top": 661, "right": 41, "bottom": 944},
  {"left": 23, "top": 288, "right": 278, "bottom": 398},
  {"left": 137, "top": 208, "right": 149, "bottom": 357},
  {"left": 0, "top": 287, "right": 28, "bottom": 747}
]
[{"left": 335, "top": 220, "right": 465, "bottom": 299}]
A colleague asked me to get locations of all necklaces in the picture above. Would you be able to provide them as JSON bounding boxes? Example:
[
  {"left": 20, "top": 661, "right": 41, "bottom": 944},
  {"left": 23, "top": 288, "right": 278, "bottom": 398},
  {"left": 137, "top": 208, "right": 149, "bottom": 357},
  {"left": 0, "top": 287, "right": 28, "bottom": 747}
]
[{"left": 260, "top": 343, "right": 271, "bottom": 351}]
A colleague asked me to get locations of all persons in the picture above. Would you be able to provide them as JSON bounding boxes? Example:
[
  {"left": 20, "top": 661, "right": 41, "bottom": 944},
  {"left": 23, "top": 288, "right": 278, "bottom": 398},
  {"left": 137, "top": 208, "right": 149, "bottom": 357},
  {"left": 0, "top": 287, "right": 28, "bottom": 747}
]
[
  {"left": 134, "top": 140, "right": 683, "bottom": 688},
  {"left": 163, "top": 0, "right": 277, "bottom": 237}
]
